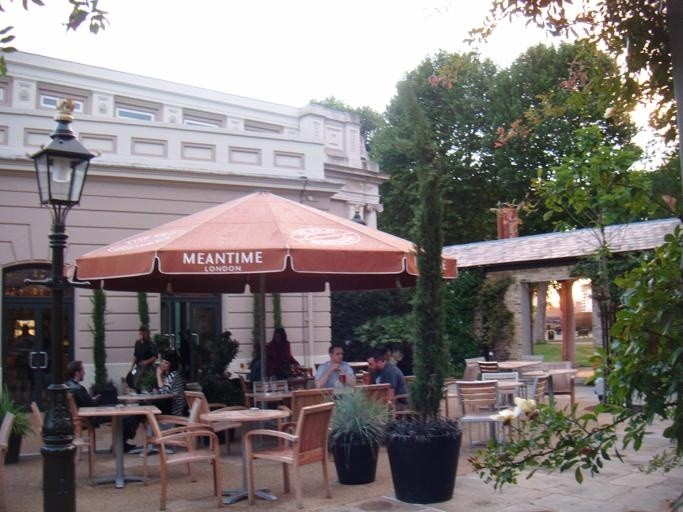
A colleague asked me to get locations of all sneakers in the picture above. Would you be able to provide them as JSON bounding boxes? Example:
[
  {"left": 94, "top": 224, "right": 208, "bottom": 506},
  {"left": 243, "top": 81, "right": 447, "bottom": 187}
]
[{"left": 109, "top": 442, "right": 178, "bottom": 457}]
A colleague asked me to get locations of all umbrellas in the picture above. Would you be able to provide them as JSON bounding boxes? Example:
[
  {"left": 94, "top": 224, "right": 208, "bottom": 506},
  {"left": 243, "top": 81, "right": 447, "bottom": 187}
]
[{"left": 73, "top": 188, "right": 458, "bottom": 445}]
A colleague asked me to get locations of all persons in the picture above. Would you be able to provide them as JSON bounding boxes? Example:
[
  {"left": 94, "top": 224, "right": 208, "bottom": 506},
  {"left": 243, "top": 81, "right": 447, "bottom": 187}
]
[
  {"left": 68, "top": 360, "right": 141, "bottom": 453},
  {"left": 314, "top": 343, "right": 356, "bottom": 388},
  {"left": 14, "top": 325, "right": 35, "bottom": 402},
  {"left": 125, "top": 328, "right": 232, "bottom": 447},
  {"left": 363, "top": 350, "right": 409, "bottom": 411},
  {"left": 267, "top": 325, "right": 299, "bottom": 377},
  {"left": 249, "top": 343, "right": 276, "bottom": 383}
]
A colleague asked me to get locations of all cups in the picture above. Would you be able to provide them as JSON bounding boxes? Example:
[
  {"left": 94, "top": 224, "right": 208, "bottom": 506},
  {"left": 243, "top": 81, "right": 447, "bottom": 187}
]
[{"left": 339, "top": 373, "right": 346, "bottom": 385}]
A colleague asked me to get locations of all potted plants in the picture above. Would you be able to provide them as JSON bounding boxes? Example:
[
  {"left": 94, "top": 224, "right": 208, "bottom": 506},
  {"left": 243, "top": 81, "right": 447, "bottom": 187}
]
[
  {"left": 383, "top": 80, "right": 466, "bottom": 505},
  {"left": 0, "top": 383, "right": 41, "bottom": 464}
]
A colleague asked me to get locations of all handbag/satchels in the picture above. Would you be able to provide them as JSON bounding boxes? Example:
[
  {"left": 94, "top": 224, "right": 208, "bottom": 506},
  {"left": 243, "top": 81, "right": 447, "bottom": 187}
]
[{"left": 126, "top": 370, "right": 145, "bottom": 389}]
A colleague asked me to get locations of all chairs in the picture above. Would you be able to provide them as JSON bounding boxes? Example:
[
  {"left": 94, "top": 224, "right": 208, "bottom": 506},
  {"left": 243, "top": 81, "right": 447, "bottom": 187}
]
[
  {"left": 67, "top": 392, "right": 88, "bottom": 464},
  {"left": 392, "top": 356, "right": 577, "bottom": 449},
  {"left": 145, "top": 408, "right": 223, "bottom": 511},
  {"left": 0, "top": 411, "right": 16, "bottom": 511},
  {"left": 184, "top": 368, "right": 390, "bottom": 508}
]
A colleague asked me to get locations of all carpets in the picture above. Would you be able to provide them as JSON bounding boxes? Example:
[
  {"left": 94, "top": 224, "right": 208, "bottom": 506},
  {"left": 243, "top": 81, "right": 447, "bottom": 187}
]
[{"left": 583, "top": 402, "right": 647, "bottom": 414}]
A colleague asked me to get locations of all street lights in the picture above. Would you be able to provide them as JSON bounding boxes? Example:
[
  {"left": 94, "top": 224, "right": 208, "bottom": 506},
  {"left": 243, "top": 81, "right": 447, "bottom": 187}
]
[{"left": 25, "top": 99, "right": 95, "bottom": 510}]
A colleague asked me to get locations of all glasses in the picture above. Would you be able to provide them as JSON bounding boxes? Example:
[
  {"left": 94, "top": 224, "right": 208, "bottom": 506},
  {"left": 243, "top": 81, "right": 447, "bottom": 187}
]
[{"left": 330, "top": 350, "right": 346, "bottom": 357}]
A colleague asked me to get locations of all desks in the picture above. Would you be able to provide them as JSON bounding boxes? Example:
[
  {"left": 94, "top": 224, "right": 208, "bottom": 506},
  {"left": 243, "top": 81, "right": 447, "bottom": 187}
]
[
  {"left": 116, "top": 393, "right": 176, "bottom": 405},
  {"left": 78, "top": 405, "right": 161, "bottom": 488}
]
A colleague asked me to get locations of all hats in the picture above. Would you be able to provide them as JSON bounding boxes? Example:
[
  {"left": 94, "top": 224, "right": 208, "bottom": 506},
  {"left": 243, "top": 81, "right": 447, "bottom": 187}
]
[{"left": 162, "top": 349, "right": 178, "bottom": 361}]
